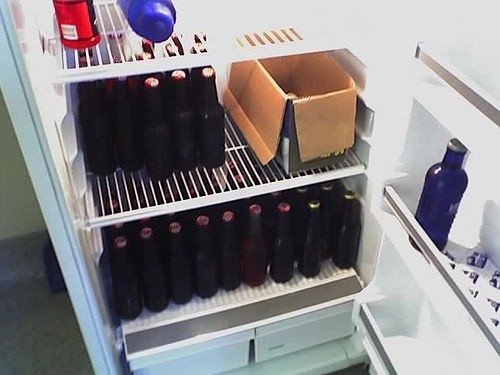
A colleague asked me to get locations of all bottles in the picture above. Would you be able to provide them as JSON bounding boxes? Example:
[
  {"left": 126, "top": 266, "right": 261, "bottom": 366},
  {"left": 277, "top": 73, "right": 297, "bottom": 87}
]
[
  {"left": 335, "top": 190, "right": 363, "bottom": 270},
  {"left": 116, "top": 0, "right": 177, "bottom": 44},
  {"left": 303, "top": 201, "right": 323, "bottom": 278},
  {"left": 138, "top": 227, "right": 170, "bottom": 313},
  {"left": 172, "top": 29, "right": 184, "bottom": 55},
  {"left": 196, "top": 67, "right": 227, "bottom": 169},
  {"left": 110, "top": 71, "right": 137, "bottom": 175},
  {"left": 165, "top": 45, "right": 177, "bottom": 58},
  {"left": 242, "top": 205, "right": 272, "bottom": 287},
  {"left": 130, "top": 38, "right": 153, "bottom": 99},
  {"left": 193, "top": 214, "right": 217, "bottom": 298},
  {"left": 108, "top": 158, "right": 283, "bottom": 212},
  {"left": 320, "top": 182, "right": 340, "bottom": 262},
  {"left": 110, "top": 222, "right": 126, "bottom": 241},
  {"left": 110, "top": 31, "right": 128, "bottom": 64},
  {"left": 54, "top": 0, "right": 100, "bottom": 47},
  {"left": 190, "top": 32, "right": 204, "bottom": 55},
  {"left": 292, "top": 186, "right": 306, "bottom": 261},
  {"left": 142, "top": 75, "right": 173, "bottom": 182},
  {"left": 169, "top": 220, "right": 195, "bottom": 303},
  {"left": 409, "top": 138, "right": 469, "bottom": 252},
  {"left": 77, "top": 49, "right": 111, "bottom": 175},
  {"left": 166, "top": 70, "right": 199, "bottom": 170},
  {"left": 111, "top": 235, "right": 143, "bottom": 321},
  {"left": 217, "top": 210, "right": 240, "bottom": 292},
  {"left": 272, "top": 202, "right": 296, "bottom": 283}
]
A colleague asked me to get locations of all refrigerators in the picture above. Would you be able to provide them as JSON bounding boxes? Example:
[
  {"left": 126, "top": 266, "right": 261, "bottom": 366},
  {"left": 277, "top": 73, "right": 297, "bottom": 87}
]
[{"left": 1, "top": 2, "right": 500, "bottom": 375}]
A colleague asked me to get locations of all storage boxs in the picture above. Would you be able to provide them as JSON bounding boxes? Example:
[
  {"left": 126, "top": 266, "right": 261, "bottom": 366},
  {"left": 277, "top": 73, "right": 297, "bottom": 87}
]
[{"left": 223, "top": 27, "right": 357, "bottom": 177}]
